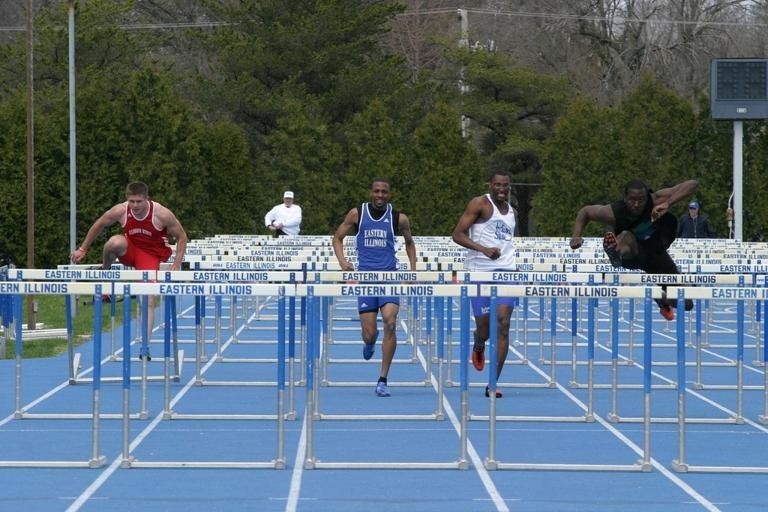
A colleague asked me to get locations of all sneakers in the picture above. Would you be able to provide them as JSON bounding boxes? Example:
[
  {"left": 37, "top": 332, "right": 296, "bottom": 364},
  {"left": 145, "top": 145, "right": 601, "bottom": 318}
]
[
  {"left": 375, "top": 381, "right": 391, "bottom": 397},
  {"left": 363, "top": 345, "right": 375, "bottom": 359},
  {"left": 604, "top": 232, "right": 622, "bottom": 268},
  {"left": 485, "top": 384, "right": 502, "bottom": 398},
  {"left": 654, "top": 295, "right": 673, "bottom": 320},
  {"left": 139, "top": 348, "right": 150, "bottom": 361},
  {"left": 93, "top": 293, "right": 112, "bottom": 302},
  {"left": 472, "top": 345, "right": 485, "bottom": 371}
]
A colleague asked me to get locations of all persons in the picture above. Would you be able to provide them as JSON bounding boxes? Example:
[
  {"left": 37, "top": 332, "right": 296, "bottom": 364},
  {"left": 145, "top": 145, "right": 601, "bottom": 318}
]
[
  {"left": 69, "top": 181, "right": 187, "bottom": 361},
  {"left": 333, "top": 178, "right": 417, "bottom": 396},
  {"left": 264, "top": 190, "right": 302, "bottom": 238},
  {"left": 569, "top": 180, "right": 700, "bottom": 320},
  {"left": 677, "top": 202, "right": 710, "bottom": 238},
  {"left": 452, "top": 170, "right": 518, "bottom": 398}
]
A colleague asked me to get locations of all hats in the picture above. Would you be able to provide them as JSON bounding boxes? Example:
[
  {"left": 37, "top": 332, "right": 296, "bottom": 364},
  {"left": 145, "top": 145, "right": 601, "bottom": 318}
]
[
  {"left": 689, "top": 202, "right": 700, "bottom": 209},
  {"left": 284, "top": 191, "right": 294, "bottom": 199}
]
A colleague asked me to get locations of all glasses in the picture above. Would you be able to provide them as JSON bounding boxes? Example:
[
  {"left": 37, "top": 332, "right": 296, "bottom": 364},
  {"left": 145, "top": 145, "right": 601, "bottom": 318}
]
[{"left": 690, "top": 207, "right": 696, "bottom": 210}]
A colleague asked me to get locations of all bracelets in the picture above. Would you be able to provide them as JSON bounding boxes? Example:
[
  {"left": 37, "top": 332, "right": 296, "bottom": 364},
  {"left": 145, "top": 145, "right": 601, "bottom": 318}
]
[{"left": 79, "top": 247, "right": 88, "bottom": 252}]
